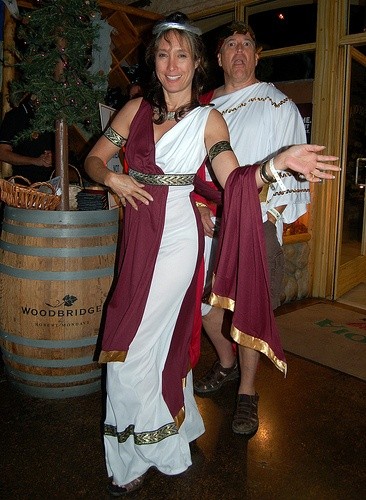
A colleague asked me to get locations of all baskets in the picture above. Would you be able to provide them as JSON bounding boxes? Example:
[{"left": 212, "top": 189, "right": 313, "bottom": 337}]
[
  {"left": 0, "top": 175, "right": 61, "bottom": 210},
  {"left": 48, "top": 164, "right": 86, "bottom": 210}
]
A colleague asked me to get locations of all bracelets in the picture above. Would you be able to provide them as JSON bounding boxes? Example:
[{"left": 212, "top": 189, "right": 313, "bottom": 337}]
[
  {"left": 195, "top": 201, "right": 208, "bottom": 208},
  {"left": 260, "top": 157, "right": 287, "bottom": 191}
]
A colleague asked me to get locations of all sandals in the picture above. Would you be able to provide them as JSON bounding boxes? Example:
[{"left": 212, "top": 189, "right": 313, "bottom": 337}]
[
  {"left": 107, "top": 472, "right": 150, "bottom": 497},
  {"left": 233, "top": 392, "right": 260, "bottom": 435},
  {"left": 192, "top": 359, "right": 240, "bottom": 393}
]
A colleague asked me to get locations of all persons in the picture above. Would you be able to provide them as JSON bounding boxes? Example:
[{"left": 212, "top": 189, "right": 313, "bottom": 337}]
[
  {"left": 0, "top": 91, "right": 57, "bottom": 187},
  {"left": 194, "top": 21, "right": 312, "bottom": 435},
  {"left": 83, "top": 11, "right": 343, "bottom": 495}
]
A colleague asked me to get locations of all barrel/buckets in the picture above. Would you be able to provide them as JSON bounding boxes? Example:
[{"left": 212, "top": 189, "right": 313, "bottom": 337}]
[{"left": 0, "top": 205, "right": 123, "bottom": 400}]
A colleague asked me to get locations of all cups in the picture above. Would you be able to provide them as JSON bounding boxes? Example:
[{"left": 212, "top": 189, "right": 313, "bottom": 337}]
[{"left": 44, "top": 150, "right": 52, "bottom": 167}]
